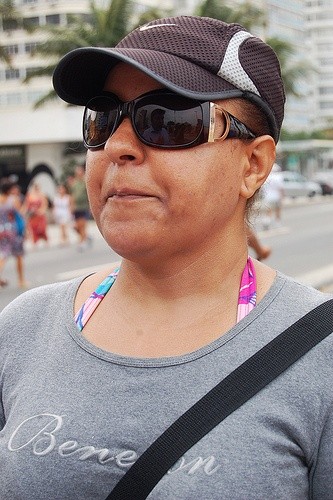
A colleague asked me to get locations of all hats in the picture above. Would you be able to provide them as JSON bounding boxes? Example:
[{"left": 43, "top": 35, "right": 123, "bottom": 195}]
[{"left": 52, "top": 15, "right": 285, "bottom": 145}]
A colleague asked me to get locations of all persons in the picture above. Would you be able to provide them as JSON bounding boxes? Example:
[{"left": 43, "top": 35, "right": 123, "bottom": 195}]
[
  {"left": 1, "top": 162, "right": 92, "bottom": 292},
  {"left": 0, "top": 14, "right": 332, "bottom": 499},
  {"left": 239, "top": 164, "right": 286, "bottom": 265}
]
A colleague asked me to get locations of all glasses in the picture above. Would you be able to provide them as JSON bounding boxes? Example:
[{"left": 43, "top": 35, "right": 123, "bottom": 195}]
[{"left": 82, "top": 88, "right": 257, "bottom": 150}]
